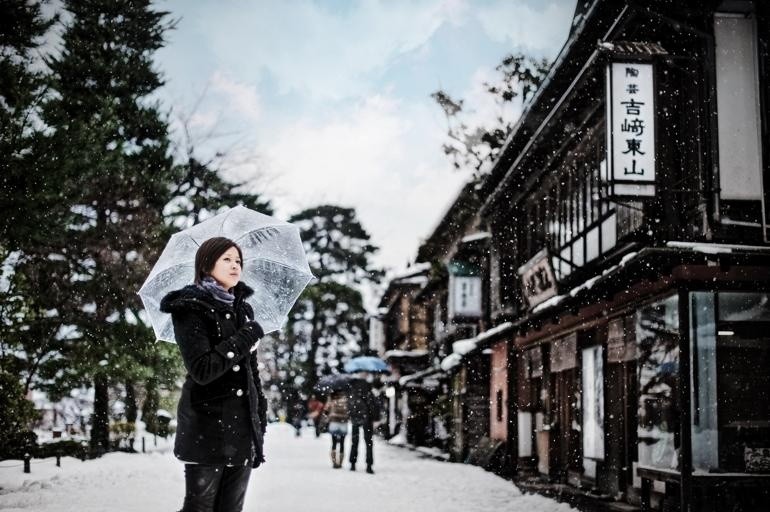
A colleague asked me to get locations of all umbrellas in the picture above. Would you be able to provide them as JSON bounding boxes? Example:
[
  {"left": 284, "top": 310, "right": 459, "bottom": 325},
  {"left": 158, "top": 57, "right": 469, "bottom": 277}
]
[
  {"left": 136, "top": 203, "right": 320, "bottom": 345},
  {"left": 342, "top": 356, "right": 392, "bottom": 374},
  {"left": 315, "top": 373, "right": 351, "bottom": 389}
]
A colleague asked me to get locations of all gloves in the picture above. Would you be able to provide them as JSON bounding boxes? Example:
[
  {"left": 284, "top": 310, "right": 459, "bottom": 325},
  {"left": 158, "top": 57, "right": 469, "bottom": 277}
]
[{"left": 245, "top": 321, "right": 264, "bottom": 346}]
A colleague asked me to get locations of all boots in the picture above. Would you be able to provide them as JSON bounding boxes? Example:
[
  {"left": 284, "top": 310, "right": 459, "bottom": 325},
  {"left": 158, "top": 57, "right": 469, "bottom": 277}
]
[
  {"left": 331, "top": 450, "right": 342, "bottom": 468},
  {"left": 338, "top": 453, "right": 344, "bottom": 468},
  {"left": 351, "top": 464, "right": 356, "bottom": 471},
  {"left": 366, "top": 463, "right": 374, "bottom": 473}
]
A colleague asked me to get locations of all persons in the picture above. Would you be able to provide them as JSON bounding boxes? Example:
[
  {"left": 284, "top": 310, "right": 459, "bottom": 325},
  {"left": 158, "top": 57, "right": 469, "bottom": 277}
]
[
  {"left": 304, "top": 393, "right": 324, "bottom": 426},
  {"left": 342, "top": 370, "right": 382, "bottom": 476},
  {"left": 157, "top": 235, "right": 269, "bottom": 511},
  {"left": 315, "top": 387, "right": 351, "bottom": 468},
  {"left": 290, "top": 397, "right": 306, "bottom": 438}
]
